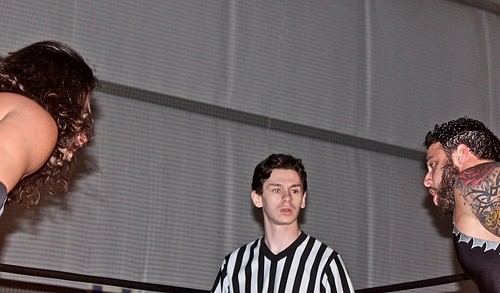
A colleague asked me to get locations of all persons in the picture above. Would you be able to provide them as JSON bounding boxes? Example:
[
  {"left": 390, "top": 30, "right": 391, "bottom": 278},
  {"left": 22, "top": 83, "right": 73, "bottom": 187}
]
[
  {"left": 423, "top": 116, "right": 500, "bottom": 293},
  {"left": 0, "top": 41, "right": 100, "bottom": 218},
  {"left": 210, "top": 153, "right": 357, "bottom": 293}
]
